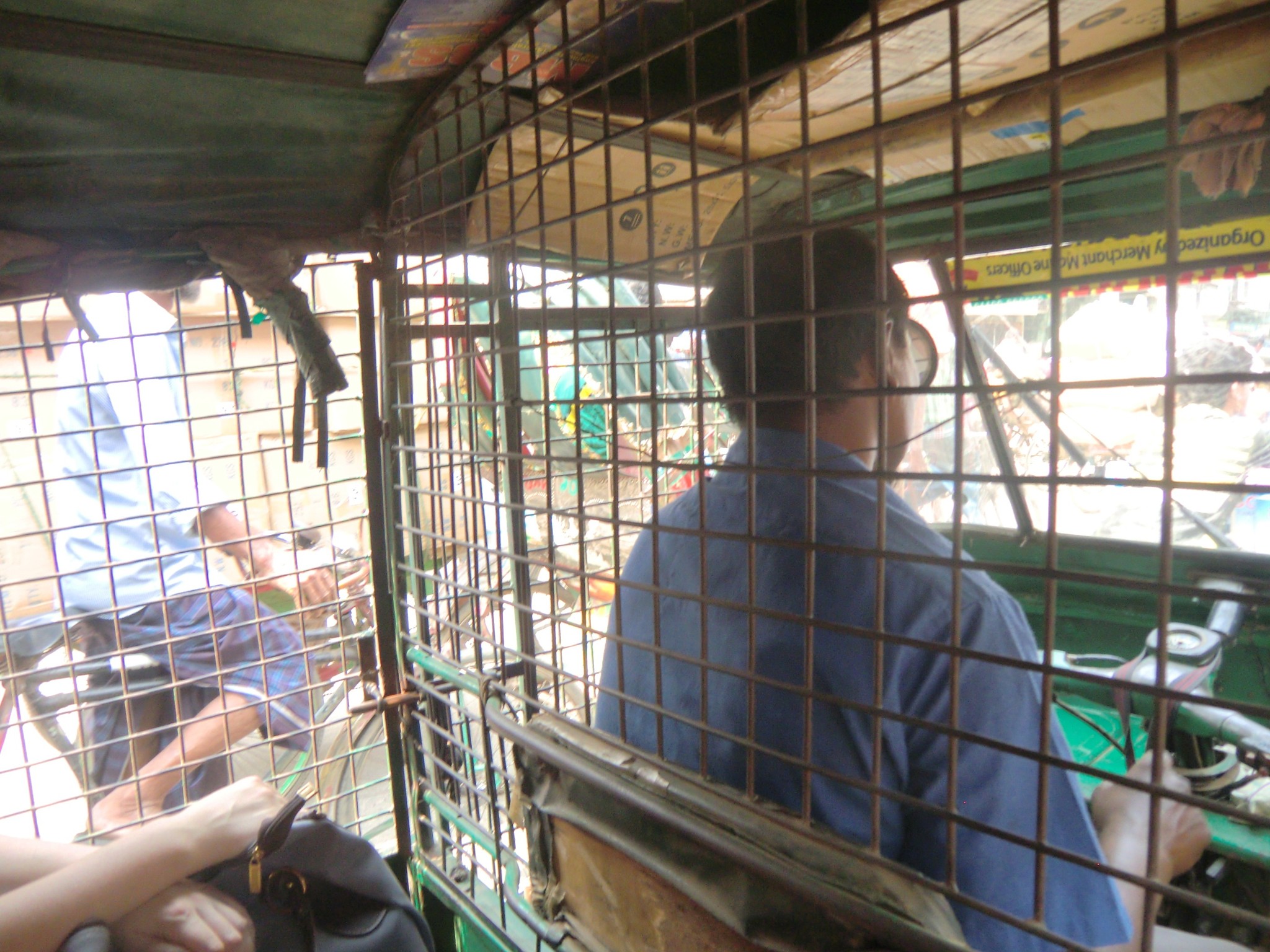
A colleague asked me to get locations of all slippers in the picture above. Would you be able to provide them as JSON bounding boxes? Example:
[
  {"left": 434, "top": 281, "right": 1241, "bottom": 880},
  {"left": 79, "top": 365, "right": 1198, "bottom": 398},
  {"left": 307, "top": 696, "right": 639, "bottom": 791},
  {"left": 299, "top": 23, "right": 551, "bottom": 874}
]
[{"left": 76, "top": 828, "right": 111, "bottom": 848}]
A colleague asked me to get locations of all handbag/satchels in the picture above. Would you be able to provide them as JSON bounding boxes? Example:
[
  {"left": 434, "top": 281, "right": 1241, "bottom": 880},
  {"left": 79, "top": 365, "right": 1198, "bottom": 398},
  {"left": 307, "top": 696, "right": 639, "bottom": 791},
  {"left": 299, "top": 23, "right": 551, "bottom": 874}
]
[{"left": 209, "top": 780, "right": 434, "bottom": 952}]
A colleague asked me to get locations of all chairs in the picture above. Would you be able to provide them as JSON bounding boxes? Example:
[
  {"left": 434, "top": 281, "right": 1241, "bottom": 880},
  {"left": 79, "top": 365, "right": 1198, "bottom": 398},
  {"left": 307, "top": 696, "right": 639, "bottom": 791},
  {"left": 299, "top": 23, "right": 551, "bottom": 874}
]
[{"left": 521, "top": 710, "right": 966, "bottom": 952}]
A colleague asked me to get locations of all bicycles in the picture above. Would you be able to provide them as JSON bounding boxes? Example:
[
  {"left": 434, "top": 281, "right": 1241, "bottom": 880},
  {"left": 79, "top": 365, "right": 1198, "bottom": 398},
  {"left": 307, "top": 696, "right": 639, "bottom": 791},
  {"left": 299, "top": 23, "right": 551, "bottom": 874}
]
[{"left": 0, "top": 530, "right": 595, "bottom": 839}]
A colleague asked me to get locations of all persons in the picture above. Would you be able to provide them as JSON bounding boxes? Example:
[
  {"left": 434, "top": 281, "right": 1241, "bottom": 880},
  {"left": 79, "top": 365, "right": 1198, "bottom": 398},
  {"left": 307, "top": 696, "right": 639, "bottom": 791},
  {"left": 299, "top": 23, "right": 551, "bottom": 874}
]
[
  {"left": 1095, "top": 341, "right": 1270, "bottom": 548},
  {"left": 0, "top": 776, "right": 309, "bottom": 952},
  {"left": 592, "top": 223, "right": 1210, "bottom": 952},
  {"left": 47, "top": 280, "right": 336, "bottom": 837}
]
